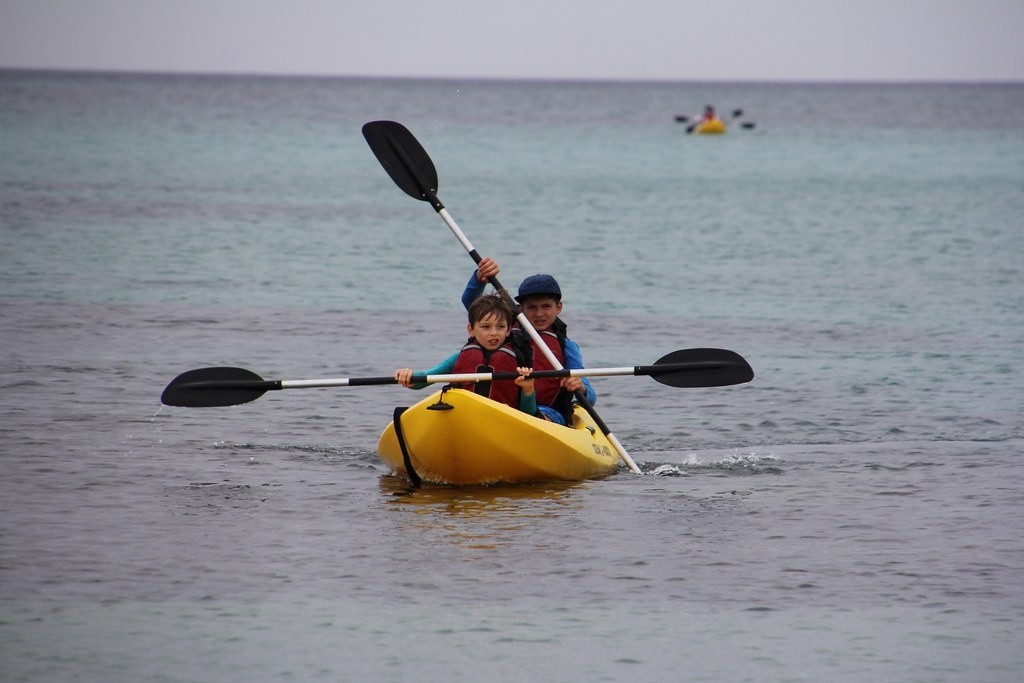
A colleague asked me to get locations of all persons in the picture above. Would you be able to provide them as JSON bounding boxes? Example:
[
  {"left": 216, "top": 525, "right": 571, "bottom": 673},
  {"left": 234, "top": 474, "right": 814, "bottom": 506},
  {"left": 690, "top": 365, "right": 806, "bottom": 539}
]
[
  {"left": 394, "top": 257, "right": 597, "bottom": 426},
  {"left": 700, "top": 104, "right": 715, "bottom": 122}
]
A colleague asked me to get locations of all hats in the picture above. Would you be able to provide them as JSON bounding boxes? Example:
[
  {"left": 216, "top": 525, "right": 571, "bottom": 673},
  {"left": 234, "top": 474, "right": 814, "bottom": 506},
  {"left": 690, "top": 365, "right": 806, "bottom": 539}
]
[{"left": 514, "top": 274, "right": 561, "bottom": 304}]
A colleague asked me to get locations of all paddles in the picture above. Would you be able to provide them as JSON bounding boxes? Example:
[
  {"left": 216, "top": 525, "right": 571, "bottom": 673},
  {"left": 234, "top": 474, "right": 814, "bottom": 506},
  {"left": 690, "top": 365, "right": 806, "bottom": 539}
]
[
  {"left": 155, "top": 347, "right": 761, "bottom": 411},
  {"left": 359, "top": 118, "right": 649, "bottom": 476},
  {"left": 673, "top": 114, "right": 756, "bottom": 131},
  {"left": 684, "top": 105, "right": 745, "bottom": 136}
]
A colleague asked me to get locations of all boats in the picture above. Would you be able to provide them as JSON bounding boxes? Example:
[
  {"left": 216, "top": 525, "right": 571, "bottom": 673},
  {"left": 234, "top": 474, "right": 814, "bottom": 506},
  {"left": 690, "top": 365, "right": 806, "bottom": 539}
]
[
  {"left": 694, "top": 117, "right": 725, "bottom": 135},
  {"left": 376, "top": 382, "right": 619, "bottom": 487}
]
